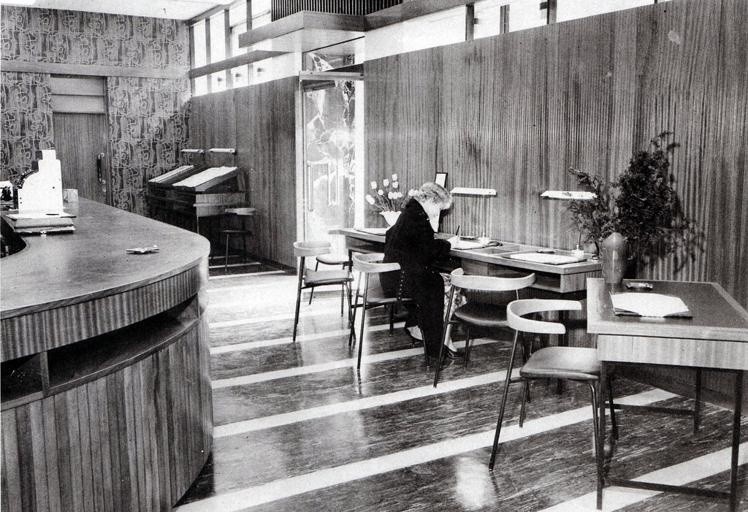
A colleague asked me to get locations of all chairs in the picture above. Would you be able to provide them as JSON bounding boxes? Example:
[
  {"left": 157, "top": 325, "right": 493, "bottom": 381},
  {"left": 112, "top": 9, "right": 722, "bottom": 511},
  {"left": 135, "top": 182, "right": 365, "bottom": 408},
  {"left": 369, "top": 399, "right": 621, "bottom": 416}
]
[
  {"left": 487, "top": 298, "right": 619, "bottom": 489},
  {"left": 290, "top": 239, "right": 359, "bottom": 341},
  {"left": 433, "top": 267, "right": 550, "bottom": 403},
  {"left": 211, "top": 207, "right": 260, "bottom": 273},
  {"left": 347, "top": 252, "right": 425, "bottom": 370}
]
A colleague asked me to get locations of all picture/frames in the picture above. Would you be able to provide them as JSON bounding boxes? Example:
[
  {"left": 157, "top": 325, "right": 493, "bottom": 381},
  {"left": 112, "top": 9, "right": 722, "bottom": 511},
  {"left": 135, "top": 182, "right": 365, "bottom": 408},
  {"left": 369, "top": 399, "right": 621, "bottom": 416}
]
[{"left": 434, "top": 172, "right": 448, "bottom": 189}]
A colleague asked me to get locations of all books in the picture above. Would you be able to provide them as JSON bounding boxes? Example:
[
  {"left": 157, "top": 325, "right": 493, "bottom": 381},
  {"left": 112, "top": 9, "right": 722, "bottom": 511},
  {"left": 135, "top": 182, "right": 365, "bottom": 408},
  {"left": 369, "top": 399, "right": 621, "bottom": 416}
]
[{"left": 453, "top": 236, "right": 503, "bottom": 251}]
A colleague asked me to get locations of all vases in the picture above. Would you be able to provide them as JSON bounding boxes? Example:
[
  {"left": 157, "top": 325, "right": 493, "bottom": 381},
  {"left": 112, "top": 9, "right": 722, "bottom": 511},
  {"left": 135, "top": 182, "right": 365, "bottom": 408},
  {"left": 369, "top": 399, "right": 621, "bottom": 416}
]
[{"left": 379, "top": 210, "right": 402, "bottom": 227}]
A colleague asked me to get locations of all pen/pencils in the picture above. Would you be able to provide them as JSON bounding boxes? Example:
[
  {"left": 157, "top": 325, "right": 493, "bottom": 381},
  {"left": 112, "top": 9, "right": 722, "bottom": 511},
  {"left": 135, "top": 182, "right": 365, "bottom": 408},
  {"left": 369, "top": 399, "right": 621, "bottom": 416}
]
[{"left": 456, "top": 225, "right": 460, "bottom": 235}]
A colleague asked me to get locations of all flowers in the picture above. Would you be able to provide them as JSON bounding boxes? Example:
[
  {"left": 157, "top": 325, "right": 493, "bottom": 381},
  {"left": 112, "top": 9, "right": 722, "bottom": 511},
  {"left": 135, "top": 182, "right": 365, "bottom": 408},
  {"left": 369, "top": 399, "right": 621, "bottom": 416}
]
[{"left": 365, "top": 173, "right": 419, "bottom": 212}]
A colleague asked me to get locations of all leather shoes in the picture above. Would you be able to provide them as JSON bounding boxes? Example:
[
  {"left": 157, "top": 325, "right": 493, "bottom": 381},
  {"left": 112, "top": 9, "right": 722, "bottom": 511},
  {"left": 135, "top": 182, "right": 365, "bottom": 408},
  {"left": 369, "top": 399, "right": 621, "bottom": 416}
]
[{"left": 442, "top": 345, "right": 462, "bottom": 359}]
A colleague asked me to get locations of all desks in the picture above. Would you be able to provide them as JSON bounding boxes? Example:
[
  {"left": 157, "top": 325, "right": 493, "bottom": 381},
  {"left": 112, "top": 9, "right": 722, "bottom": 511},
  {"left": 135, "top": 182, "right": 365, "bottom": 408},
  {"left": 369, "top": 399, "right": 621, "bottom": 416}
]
[
  {"left": 455, "top": 239, "right": 602, "bottom": 393},
  {"left": 339, "top": 227, "right": 492, "bottom": 301},
  {"left": 584, "top": 277, "right": 748, "bottom": 511}
]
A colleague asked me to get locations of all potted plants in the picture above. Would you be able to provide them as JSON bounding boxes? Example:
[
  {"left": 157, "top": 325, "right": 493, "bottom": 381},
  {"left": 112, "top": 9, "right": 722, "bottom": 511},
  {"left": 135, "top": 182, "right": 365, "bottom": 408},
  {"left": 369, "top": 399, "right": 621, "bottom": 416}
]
[{"left": 567, "top": 128, "right": 707, "bottom": 286}]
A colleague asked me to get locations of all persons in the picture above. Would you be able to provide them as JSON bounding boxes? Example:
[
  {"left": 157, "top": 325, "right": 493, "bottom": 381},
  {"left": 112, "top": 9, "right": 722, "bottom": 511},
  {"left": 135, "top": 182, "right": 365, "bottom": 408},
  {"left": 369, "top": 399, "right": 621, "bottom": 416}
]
[{"left": 379, "top": 182, "right": 468, "bottom": 367}]
